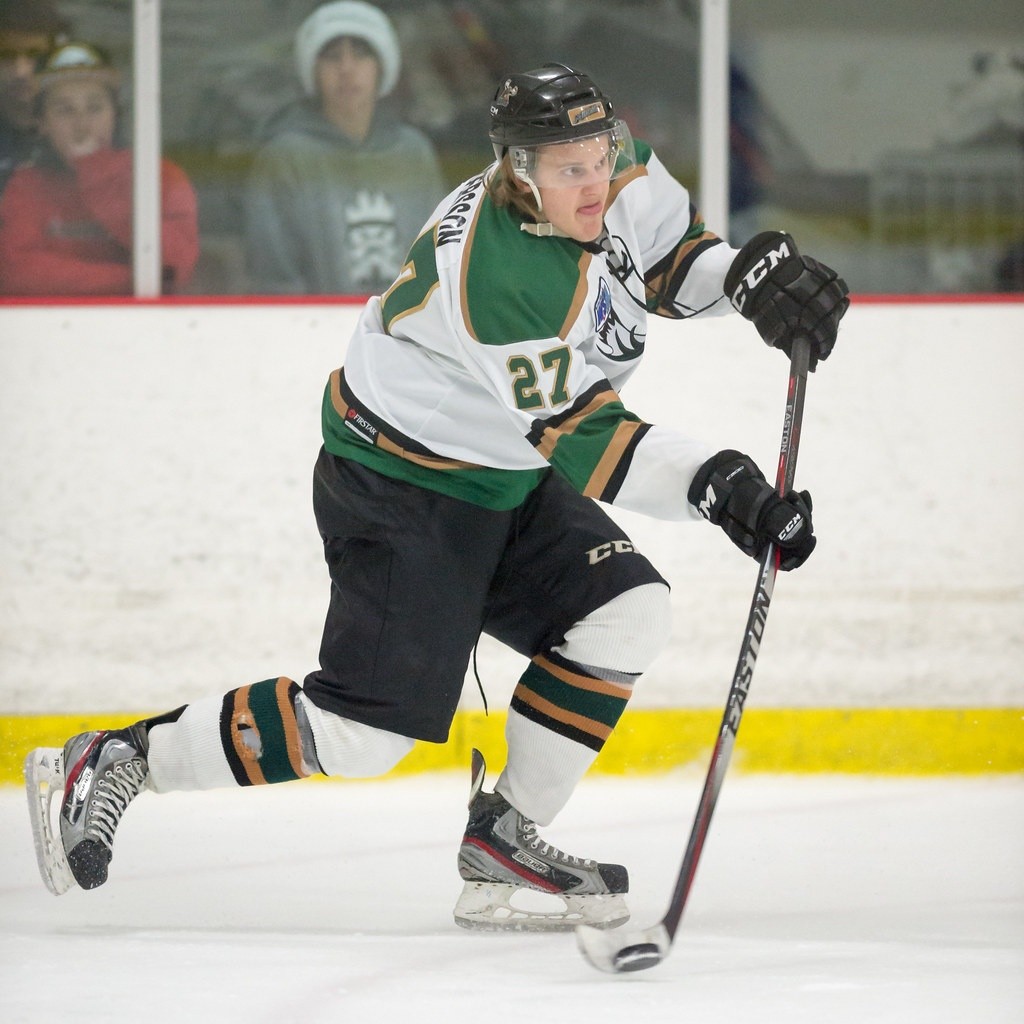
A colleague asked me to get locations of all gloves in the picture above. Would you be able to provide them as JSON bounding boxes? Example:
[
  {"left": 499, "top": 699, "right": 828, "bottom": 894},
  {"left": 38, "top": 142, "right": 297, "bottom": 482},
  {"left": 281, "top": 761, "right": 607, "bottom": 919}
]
[
  {"left": 688, "top": 450, "right": 816, "bottom": 572},
  {"left": 722, "top": 230, "right": 850, "bottom": 372}
]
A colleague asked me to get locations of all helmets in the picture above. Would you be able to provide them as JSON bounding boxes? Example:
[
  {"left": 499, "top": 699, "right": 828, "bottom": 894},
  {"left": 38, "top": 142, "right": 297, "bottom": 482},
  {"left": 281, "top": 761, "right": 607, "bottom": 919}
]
[{"left": 488, "top": 63, "right": 620, "bottom": 149}]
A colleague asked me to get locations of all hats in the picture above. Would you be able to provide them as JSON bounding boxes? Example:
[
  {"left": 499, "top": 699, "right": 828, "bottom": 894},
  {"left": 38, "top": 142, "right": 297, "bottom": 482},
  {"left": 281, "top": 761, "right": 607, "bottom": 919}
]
[
  {"left": 290, "top": 0, "right": 404, "bottom": 98},
  {"left": 27, "top": 33, "right": 119, "bottom": 96}
]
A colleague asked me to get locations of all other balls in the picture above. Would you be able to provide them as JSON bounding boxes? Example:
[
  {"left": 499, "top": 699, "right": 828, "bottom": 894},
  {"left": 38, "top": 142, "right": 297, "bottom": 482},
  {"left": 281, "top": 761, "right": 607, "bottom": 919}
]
[{"left": 608, "top": 939, "right": 667, "bottom": 976}]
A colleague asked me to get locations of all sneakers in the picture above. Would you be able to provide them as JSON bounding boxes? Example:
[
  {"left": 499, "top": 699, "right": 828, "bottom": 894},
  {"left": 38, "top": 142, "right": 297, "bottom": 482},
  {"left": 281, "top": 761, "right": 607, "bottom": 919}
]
[
  {"left": 453, "top": 747, "right": 629, "bottom": 935},
  {"left": 24, "top": 704, "right": 188, "bottom": 894}
]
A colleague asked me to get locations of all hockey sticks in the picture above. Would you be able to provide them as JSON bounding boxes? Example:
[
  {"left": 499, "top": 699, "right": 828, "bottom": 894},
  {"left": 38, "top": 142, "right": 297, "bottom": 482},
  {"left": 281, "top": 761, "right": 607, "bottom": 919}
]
[{"left": 571, "top": 326, "right": 816, "bottom": 979}]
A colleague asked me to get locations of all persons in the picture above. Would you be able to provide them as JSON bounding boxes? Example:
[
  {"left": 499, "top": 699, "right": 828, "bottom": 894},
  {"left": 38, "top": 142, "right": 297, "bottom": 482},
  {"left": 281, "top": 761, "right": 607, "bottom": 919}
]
[
  {"left": 0, "top": 0, "right": 792, "bottom": 296},
  {"left": 26, "top": 62, "right": 851, "bottom": 933}
]
